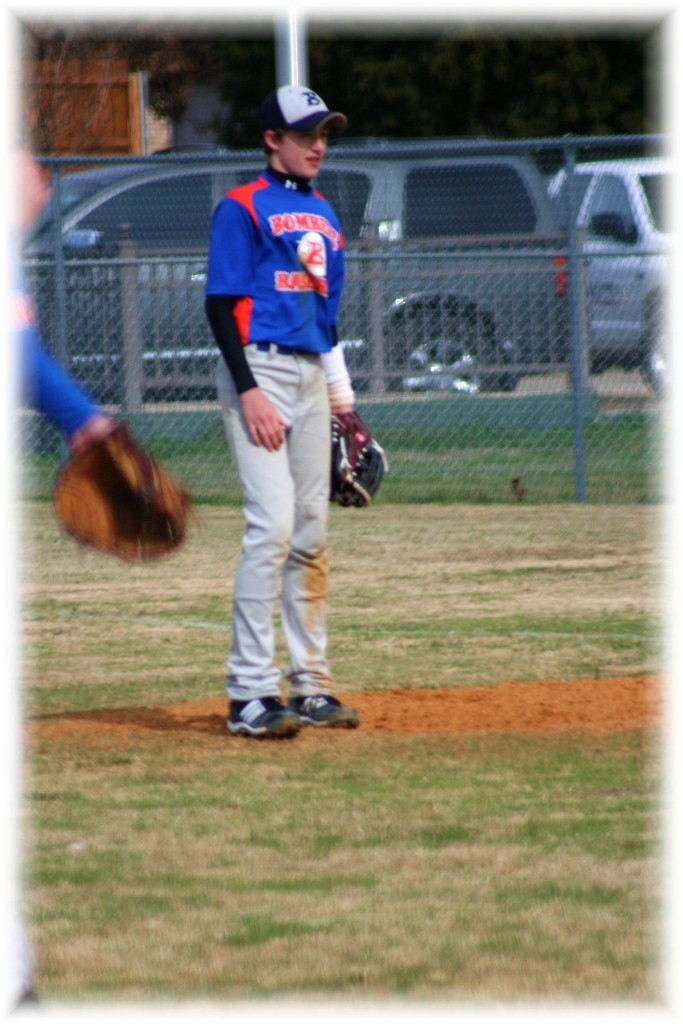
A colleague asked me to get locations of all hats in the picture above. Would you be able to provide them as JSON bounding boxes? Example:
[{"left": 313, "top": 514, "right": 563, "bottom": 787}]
[{"left": 263, "top": 85, "right": 348, "bottom": 134}]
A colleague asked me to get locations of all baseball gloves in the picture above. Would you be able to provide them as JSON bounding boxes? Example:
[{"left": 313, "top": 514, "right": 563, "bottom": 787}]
[
  {"left": 330, "top": 416, "right": 388, "bottom": 510},
  {"left": 54, "top": 419, "right": 191, "bottom": 564}
]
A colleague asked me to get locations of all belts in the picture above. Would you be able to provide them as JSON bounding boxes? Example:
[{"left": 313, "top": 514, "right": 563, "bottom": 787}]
[{"left": 257, "top": 339, "right": 304, "bottom": 355}]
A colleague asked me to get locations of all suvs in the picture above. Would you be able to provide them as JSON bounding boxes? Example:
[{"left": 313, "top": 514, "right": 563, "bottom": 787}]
[{"left": 22, "top": 142, "right": 568, "bottom": 403}]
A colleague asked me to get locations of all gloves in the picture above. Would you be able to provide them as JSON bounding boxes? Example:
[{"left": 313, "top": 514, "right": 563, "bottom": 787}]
[{"left": 337, "top": 408, "right": 371, "bottom": 474}]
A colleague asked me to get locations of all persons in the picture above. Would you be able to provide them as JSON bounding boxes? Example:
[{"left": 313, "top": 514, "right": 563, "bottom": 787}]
[
  {"left": 11, "top": 143, "right": 114, "bottom": 460},
  {"left": 205, "top": 85, "right": 387, "bottom": 739}
]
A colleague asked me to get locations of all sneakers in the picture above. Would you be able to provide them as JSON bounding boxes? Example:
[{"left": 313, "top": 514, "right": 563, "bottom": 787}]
[
  {"left": 288, "top": 695, "right": 359, "bottom": 729},
  {"left": 226, "top": 697, "right": 302, "bottom": 738}
]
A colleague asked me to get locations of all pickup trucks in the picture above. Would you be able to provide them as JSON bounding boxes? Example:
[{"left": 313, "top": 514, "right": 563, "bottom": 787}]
[{"left": 545, "top": 155, "right": 668, "bottom": 398}]
